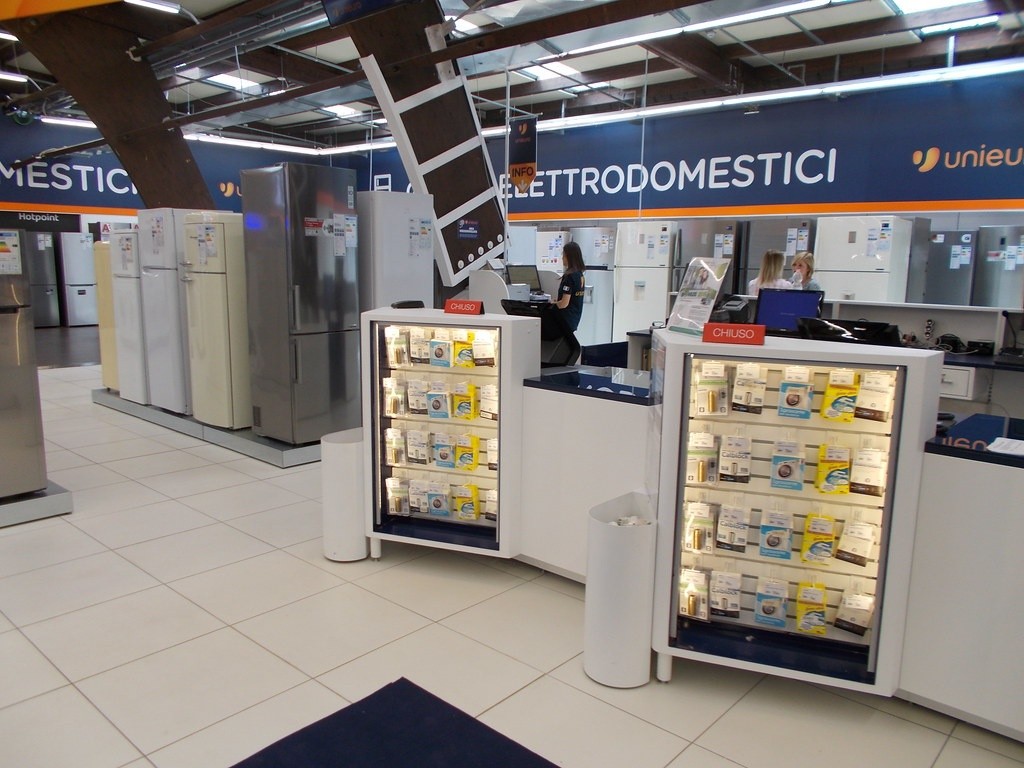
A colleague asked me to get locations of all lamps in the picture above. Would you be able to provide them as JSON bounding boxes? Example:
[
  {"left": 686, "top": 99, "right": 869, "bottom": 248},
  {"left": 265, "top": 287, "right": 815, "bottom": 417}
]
[
  {"left": 0, "top": 71, "right": 28, "bottom": 82},
  {"left": 0, "top": 29, "right": 19, "bottom": 41},
  {"left": 512, "top": 70, "right": 538, "bottom": 82},
  {"left": 40, "top": 115, "right": 97, "bottom": 128},
  {"left": 124, "top": 0, "right": 180, "bottom": 14}
]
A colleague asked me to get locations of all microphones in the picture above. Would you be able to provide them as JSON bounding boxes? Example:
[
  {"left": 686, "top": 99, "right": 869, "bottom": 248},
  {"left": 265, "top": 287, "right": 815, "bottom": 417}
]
[{"left": 1002, "top": 310, "right": 1017, "bottom": 352}]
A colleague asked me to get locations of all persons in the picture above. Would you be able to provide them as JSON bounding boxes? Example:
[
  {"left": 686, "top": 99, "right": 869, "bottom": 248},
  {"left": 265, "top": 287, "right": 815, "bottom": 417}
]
[
  {"left": 552, "top": 241, "right": 586, "bottom": 332},
  {"left": 788, "top": 251, "right": 822, "bottom": 290},
  {"left": 748, "top": 249, "right": 793, "bottom": 296}
]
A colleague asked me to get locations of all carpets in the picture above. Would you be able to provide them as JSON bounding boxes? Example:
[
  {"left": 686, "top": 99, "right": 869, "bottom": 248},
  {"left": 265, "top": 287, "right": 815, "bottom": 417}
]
[{"left": 230, "top": 677, "right": 562, "bottom": 768}]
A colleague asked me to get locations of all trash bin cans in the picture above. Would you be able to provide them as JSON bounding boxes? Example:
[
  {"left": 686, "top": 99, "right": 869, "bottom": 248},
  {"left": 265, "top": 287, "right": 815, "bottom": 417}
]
[
  {"left": 583, "top": 492, "right": 660, "bottom": 689},
  {"left": 320, "top": 427, "right": 369, "bottom": 563}
]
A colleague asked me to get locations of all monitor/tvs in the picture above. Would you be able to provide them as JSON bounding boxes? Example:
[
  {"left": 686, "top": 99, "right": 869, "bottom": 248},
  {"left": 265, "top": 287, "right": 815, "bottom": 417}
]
[
  {"left": 797, "top": 317, "right": 901, "bottom": 347},
  {"left": 720, "top": 293, "right": 761, "bottom": 329},
  {"left": 752, "top": 288, "right": 825, "bottom": 339},
  {"left": 506, "top": 264, "right": 541, "bottom": 292},
  {"left": 500, "top": 298, "right": 582, "bottom": 367}
]
